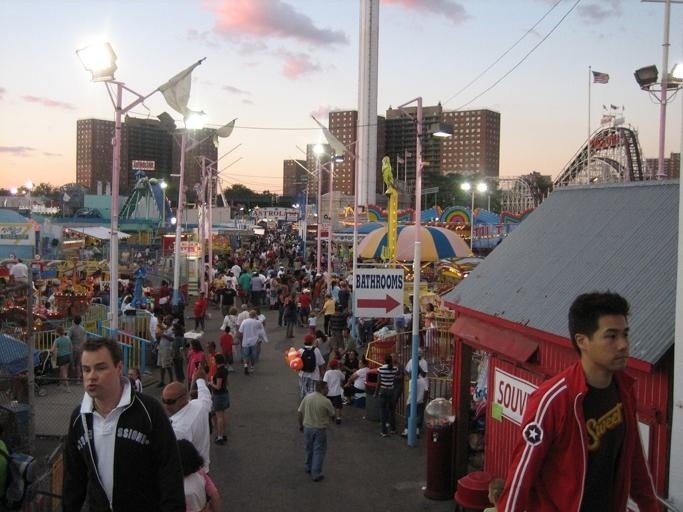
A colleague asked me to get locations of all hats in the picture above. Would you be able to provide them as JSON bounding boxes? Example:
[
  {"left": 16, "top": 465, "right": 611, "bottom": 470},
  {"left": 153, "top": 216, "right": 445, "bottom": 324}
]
[
  {"left": 330, "top": 360, "right": 338, "bottom": 366},
  {"left": 304, "top": 335, "right": 313, "bottom": 342}
]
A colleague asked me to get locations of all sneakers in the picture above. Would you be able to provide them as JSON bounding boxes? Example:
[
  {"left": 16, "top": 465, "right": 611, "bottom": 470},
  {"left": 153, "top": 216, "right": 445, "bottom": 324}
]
[
  {"left": 215, "top": 436, "right": 226, "bottom": 444},
  {"left": 314, "top": 476, "right": 324, "bottom": 481},
  {"left": 336, "top": 419, "right": 341, "bottom": 424}
]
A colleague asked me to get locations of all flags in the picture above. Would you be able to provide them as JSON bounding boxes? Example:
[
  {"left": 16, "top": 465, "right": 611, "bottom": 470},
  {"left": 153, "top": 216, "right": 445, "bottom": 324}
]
[
  {"left": 592, "top": 71, "right": 609, "bottom": 84},
  {"left": 212, "top": 120, "right": 235, "bottom": 148},
  {"left": 157, "top": 60, "right": 207, "bottom": 120},
  {"left": 317, "top": 122, "right": 346, "bottom": 156}
]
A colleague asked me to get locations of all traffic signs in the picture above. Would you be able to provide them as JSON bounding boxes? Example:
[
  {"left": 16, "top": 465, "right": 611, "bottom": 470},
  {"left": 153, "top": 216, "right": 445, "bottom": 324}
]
[{"left": 353, "top": 268, "right": 403, "bottom": 319}]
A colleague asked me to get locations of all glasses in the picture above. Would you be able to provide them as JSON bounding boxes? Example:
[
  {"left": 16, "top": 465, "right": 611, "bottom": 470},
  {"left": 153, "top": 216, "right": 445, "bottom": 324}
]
[{"left": 162, "top": 394, "right": 183, "bottom": 404}]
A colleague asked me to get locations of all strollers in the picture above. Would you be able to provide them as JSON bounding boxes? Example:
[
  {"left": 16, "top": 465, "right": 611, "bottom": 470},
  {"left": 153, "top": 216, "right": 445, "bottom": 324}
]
[{"left": 9, "top": 349, "right": 51, "bottom": 399}]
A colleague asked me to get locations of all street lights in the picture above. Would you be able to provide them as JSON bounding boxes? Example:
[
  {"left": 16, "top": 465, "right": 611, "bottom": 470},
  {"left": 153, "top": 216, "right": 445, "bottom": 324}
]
[
  {"left": 311, "top": 143, "right": 323, "bottom": 275},
  {"left": 396, "top": 97, "right": 455, "bottom": 447},
  {"left": 159, "top": 180, "right": 166, "bottom": 227},
  {"left": 634, "top": 61, "right": 683, "bottom": 179},
  {"left": 459, "top": 182, "right": 488, "bottom": 253},
  {"left": 73, "top": 40, "right": 123, "bottom": 335},
  {"left": 326, "top": 154, "right": 345, "bottom": 284},
  {"left": 172, "top": 107, "right": 206, "bottom": 306},
  {"left": 10, "top": 182, "right": 33, "bottom": 210}
]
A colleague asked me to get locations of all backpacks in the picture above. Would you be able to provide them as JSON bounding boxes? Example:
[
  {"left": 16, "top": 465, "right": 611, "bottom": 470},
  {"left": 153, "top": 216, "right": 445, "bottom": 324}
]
[
  {"left": 1, "top": 450, "right": 38, "bottom": 512},
  {"left": 302, "top": 347, "right": 316, "bottom": 372}
]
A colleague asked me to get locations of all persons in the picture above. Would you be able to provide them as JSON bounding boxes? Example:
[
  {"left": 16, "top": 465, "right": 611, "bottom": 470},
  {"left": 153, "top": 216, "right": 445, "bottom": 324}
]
[
  {"left": 178, "top": 438, "right": 224, "bottom": 512},
  {"left": 496, "top": 288, "right": 659, "bottom": 512},
  {"left": 297, "top": 381, "right": 335, "bottom": 482},
  {"left": 61, "top": 336, "right": 188, "bottom": 511},
  {"left": 0, "top": 441, "right": 22, "bottom": 512},
  {"left": 1, "top": 222, "right": 445, "bottom": 445},
  {"left": 483, "top": 477, "right": 504, "bottom": 512},
  {"left": 162, "top": 362, "right": 215, "bottom": 502}
]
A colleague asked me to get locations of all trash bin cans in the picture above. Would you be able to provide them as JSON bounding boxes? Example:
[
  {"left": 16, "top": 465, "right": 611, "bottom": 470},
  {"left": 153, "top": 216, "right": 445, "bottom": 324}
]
[{"left": 364, "top": 368, "right": 380, "bottom": 421}]
